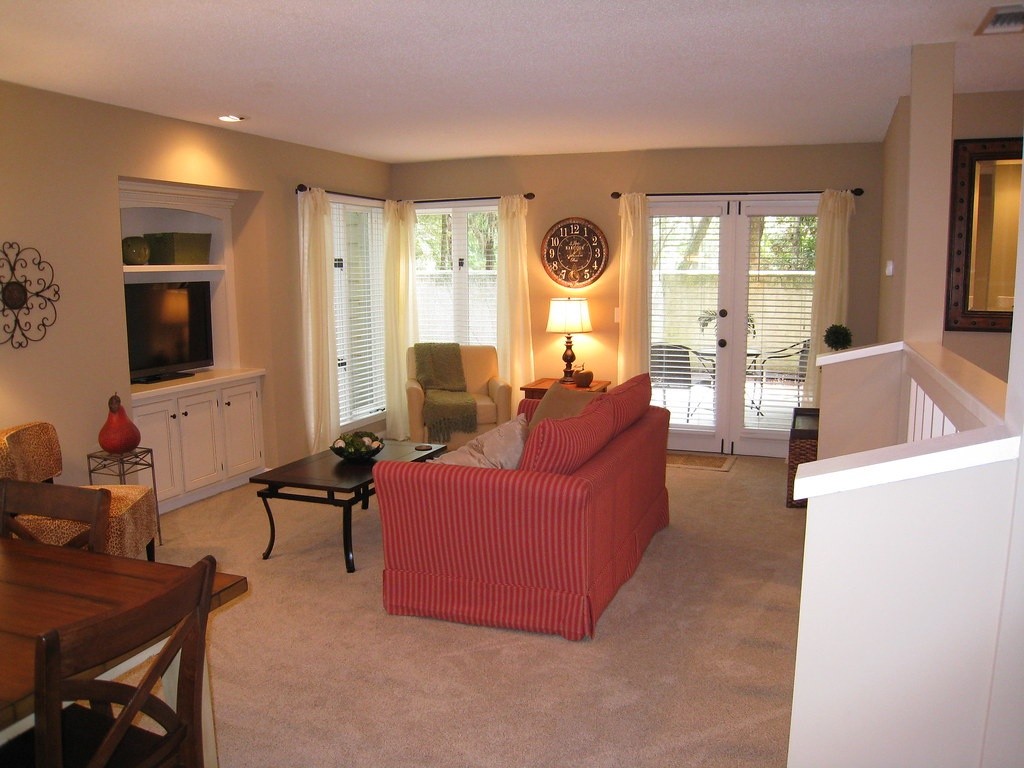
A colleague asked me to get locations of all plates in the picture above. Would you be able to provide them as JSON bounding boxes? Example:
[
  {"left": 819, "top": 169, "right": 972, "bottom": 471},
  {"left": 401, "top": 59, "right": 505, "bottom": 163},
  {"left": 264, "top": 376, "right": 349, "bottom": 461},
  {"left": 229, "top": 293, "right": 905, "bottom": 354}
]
[{"left": 329, "top": 443, "right": 385, "bottom": 459}]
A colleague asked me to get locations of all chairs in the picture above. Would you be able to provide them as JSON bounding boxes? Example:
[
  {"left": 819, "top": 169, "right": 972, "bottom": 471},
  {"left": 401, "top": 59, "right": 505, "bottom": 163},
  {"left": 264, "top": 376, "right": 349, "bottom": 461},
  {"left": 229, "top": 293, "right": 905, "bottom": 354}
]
[
  {"left": 0, "top": 477, "right": 113, "bottom": 556},
  {"left": 752, "top": 339, "right": 809, "bottom": 415},
  {"left": 0, "top": 422, "right": 159, "bottom": 558},
  {"left": 649, "top": 343, "right": 702, "bottom": 423},
  {"left": 0, "top": 555, "right": 217, "bottom": 768},
  {"left": 406, "top": 342, "right": 513, "bottom": 450}
]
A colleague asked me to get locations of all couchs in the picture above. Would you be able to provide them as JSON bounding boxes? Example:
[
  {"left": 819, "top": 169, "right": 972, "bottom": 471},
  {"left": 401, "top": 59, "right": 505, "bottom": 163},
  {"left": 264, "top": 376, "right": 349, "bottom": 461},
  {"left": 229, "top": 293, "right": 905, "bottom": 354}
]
[{"left": 372, "top": 369, "right": 671, "bottom": 643}]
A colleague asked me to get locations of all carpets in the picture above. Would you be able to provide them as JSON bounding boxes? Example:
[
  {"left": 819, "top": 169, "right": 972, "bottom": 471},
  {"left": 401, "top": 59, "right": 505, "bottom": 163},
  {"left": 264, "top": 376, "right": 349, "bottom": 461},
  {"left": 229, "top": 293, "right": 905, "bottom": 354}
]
[{"left": 665, "top": 449, "right": 738, "bottom": 472}]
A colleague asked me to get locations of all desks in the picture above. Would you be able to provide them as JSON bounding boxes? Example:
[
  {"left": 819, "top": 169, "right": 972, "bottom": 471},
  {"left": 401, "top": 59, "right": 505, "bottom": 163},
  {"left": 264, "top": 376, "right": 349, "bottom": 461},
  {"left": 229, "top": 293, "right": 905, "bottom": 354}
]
[
  {"left": 520, "top": 378, "right": 611, "bottom": 400},
  {"left": 250, "top": 440, "right": 446, "bottom": 575},
  {"left": 87, "top": 445, "right": 164, "bottom": 546},
  {"left": 691, "top": 348, "right": 765, "bottom": 419},
  {"left": 0, "top": 533, "right": 248, "bottom": 768}
]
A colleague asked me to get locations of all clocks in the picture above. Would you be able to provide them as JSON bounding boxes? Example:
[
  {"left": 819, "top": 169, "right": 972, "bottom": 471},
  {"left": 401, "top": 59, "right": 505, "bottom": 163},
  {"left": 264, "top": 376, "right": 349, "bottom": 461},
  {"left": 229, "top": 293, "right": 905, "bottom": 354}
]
[{"left": 539, "top": 217, "right": 610, "bottom": 289}]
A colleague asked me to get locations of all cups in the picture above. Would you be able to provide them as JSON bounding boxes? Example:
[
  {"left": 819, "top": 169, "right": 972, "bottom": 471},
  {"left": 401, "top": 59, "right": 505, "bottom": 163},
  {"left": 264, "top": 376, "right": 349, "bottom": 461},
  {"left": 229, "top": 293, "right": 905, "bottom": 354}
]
[{"left": 574, "top": 371, "right": 593, "bottom": 386}]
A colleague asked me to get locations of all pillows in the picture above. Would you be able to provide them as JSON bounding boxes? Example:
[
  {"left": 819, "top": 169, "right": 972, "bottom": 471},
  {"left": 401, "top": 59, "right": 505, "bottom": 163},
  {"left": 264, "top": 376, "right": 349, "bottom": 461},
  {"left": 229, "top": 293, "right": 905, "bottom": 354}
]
[{"left": 432, "top": 373, "right": 652, "bottom": 478}]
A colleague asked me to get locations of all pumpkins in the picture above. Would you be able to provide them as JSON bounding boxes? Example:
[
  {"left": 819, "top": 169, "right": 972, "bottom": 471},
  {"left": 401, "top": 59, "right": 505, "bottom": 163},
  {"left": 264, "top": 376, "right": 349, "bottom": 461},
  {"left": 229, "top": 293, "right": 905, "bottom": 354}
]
[
  {"left": 98, "top": 392, "right": 141, "bottom": 453},
  {"left": 572, "top": 362, "right": 593, "bottom": 387}
]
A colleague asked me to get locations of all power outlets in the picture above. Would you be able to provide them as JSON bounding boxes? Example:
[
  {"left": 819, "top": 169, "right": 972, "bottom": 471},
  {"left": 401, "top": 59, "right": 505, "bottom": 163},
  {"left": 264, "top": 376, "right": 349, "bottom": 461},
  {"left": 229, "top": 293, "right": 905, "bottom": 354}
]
[{"left": 885, "top": 260, "right": 893, "bottom": 278}]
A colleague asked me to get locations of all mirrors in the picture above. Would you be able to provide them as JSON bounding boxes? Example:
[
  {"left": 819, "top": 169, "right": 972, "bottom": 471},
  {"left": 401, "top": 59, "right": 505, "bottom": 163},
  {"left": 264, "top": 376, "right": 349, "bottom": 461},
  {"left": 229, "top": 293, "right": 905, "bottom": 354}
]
[{"left": 942, "top": 138, "right": 1024, "bottom": 333}]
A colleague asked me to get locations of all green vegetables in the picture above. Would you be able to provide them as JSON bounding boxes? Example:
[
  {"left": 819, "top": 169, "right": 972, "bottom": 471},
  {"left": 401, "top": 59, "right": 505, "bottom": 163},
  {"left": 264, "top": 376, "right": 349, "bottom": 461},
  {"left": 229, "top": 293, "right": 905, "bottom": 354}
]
[{"left": 340, "top": 431, "right": 383, "bottom": 444}]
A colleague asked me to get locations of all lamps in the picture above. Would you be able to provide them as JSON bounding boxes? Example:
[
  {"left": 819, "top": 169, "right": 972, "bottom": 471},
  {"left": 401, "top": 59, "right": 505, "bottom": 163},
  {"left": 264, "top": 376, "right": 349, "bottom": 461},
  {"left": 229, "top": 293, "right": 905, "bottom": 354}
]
[{"left": 545, "top": 296, "right": 594, "bottom": 385}]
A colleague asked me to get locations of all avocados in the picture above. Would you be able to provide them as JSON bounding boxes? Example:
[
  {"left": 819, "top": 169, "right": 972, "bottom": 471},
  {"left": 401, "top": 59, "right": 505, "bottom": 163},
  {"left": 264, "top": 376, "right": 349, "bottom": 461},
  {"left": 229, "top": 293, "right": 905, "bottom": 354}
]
[{"left": 346, "top": 438, "right": 371, "bottom": 453}]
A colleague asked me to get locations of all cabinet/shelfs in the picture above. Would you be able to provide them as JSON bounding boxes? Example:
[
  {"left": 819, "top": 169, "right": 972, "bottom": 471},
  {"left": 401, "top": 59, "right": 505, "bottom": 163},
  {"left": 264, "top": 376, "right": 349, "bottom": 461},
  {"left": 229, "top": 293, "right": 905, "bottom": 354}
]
[
  {"left": 131, "top": 366, "right": 271, "bottom": 515},
  {"left": 787, "top": 408, "right": 820, "bottom": 508}
]
